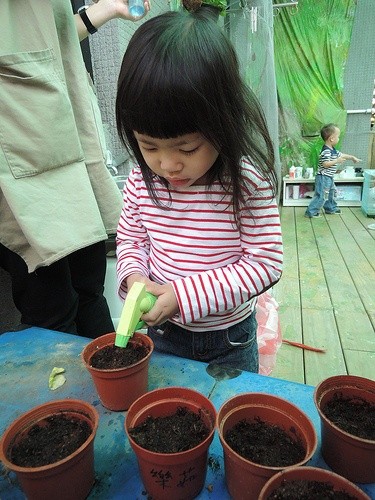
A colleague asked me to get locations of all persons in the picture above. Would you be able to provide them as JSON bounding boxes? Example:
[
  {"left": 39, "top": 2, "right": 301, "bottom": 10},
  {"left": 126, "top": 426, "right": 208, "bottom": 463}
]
[
  {"left": 115, "top": 11, "right": 283, "bottom": 374},
  {"left": 0, "top": 0, "right": 150, "bottom": 340},
  {"left": 304, "top": 123, "right": 363, "bottom": 218}
]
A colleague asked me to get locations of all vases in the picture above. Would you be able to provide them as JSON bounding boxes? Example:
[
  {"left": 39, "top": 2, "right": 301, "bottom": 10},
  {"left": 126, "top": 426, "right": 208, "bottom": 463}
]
[
  {"left": 217, "top": 393, "right": 318, "bottom": 500},
  {"left": 0, "top": 398, "right": 100, "bottom": 500},
  {"left": 82, "top": 332, "right": 154, "bottom": 411},
  {"left": 259, "top": 467, "right": 372, "bottom": 500},
  {"left": 313, "top": 374, "right": 375, "bottom": 486},
  {"left": 126, "top": 388, "right": 217, "bottom": 500}
]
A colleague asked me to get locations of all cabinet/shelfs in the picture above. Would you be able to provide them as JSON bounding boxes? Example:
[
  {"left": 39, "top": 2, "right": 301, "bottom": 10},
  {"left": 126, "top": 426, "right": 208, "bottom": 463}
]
[{"left": 283, "top": 177, "right": 363, "bottom": 207}]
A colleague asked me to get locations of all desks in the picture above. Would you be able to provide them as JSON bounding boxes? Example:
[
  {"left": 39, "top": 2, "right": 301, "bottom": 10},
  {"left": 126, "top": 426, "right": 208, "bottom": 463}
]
[{"left": 0, "top": 325, "right": 375, "bottom": 500}]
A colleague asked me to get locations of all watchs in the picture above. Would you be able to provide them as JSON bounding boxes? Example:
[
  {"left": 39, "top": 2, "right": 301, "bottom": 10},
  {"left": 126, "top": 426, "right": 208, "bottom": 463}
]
[{"left": 77, "top": 5, "right": 98, "bottom": 35}]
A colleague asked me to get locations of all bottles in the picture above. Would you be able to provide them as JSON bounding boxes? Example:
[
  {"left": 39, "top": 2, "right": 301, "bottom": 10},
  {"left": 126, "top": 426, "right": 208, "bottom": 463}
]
[{"left": 129, "top": 0, "right": 145, "bottom": 19}]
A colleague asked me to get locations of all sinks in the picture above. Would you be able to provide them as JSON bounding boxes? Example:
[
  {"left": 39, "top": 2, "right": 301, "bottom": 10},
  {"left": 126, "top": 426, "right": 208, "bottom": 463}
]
[{"left": 113, "top": 175, "right": 128, "bottom": 191}]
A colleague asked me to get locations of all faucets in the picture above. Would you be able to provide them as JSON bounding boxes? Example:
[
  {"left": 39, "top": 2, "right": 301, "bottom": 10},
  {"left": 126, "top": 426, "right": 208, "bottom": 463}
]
[{"left": 104, "top": 151, "right": 118, "bottom": 175}]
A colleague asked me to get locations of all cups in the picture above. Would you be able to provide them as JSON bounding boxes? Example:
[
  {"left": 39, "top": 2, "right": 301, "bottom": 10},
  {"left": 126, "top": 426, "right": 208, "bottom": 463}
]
[
  {"left": 289, "top": 167, "right": 302, "bottom": 180},
  {"left": 293, "top": 186, "right": 299, "bottom": 199}
]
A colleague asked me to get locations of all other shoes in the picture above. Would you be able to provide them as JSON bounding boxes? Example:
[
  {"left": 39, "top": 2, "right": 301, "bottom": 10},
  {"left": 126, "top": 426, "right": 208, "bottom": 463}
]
[
  {"left": 330, "top": 209, "right": 342, "bottom": 215},
  {"left": 313, "top": 213, "right": 322, "bottom": 219}
]
